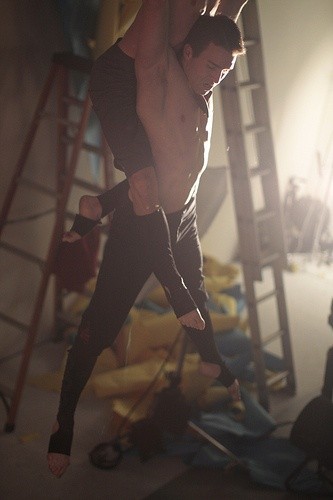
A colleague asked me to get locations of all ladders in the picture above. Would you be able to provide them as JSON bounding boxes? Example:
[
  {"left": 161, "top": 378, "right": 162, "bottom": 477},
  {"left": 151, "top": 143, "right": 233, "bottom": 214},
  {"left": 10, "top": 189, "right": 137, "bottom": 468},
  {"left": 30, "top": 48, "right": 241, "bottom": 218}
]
[
  {"left": 218, "top": 0, "right": 297, "bottom": 411},
  {"left": 1, "top": 52, "right": 130, "bottom": 435}
]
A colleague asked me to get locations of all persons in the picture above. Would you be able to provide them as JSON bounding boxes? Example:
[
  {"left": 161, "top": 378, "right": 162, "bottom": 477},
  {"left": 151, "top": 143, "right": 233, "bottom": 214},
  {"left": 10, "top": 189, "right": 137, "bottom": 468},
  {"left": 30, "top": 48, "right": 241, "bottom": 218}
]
[
  {"left": 58, "top": 1, "right": 209, "bottom": 332},
  {"left": 47, "top": 0, "right": 250, "bottom": 479}
]
